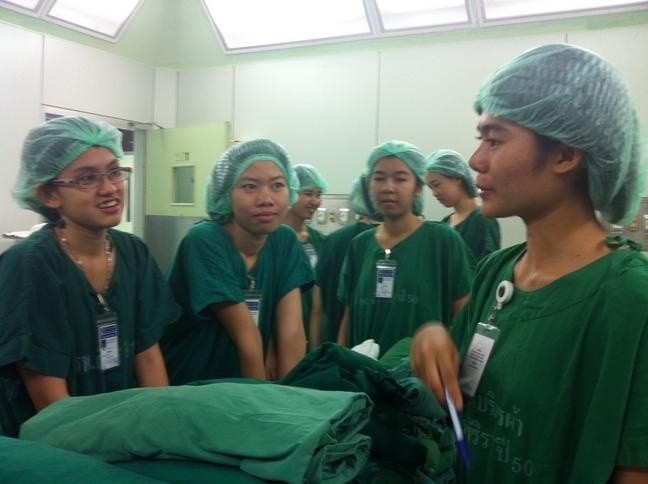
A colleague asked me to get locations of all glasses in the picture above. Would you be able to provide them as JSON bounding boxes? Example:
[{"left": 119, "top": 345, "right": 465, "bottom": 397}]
[{"left": 50, "top": 167, "right": 132, "bottom": 189}]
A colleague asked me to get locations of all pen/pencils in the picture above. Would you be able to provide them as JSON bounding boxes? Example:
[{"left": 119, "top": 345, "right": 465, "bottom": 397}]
[{"left": 444, "top": 384, "right": 469, "bottom": 460}]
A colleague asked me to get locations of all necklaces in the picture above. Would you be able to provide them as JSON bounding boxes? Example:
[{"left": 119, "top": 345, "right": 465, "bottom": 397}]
[{"left": 55, "top": 220, "right": 113, "bottom": 292}]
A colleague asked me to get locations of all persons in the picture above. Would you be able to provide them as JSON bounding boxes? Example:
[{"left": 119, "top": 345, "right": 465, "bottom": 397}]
[
  {"left": 412, "top": 44, "right": 648, "bottom": 481},
  {"left": 279, "top": 165, "right": 328, "bottom": 342},
  {"left": 1, "top": 119, "right": 170, "bottom": 435},
  {"left": 425, "top": 150, "right": 502, "bottom": 265},
  {"left": 338, "top": 138, "right": 472, "bottom": 379},
  {"left": 161, "top": 140, "right": 314, "bottom": 386},
  {"left": 309, "top": 168, "right": 424, "bottom": 352}
]
